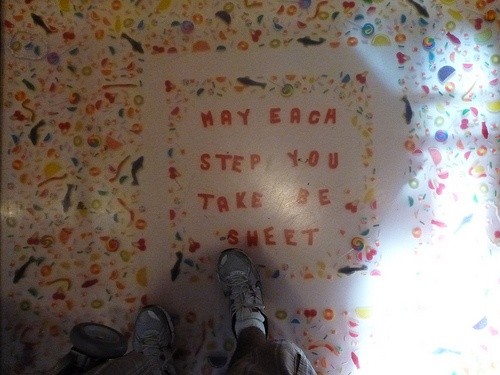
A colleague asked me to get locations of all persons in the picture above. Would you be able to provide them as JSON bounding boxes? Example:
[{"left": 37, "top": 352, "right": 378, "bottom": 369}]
[{"left": 80, "top": 246, "right": 322, "bottom": 375}]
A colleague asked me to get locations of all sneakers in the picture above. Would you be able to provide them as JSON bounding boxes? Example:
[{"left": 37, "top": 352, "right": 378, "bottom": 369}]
[
  {"left": 217, "top": 247, "right": 268, "bottom": 341},
  {"left": 132, "top": 303, "right": 174, "bottom": 375}
]
